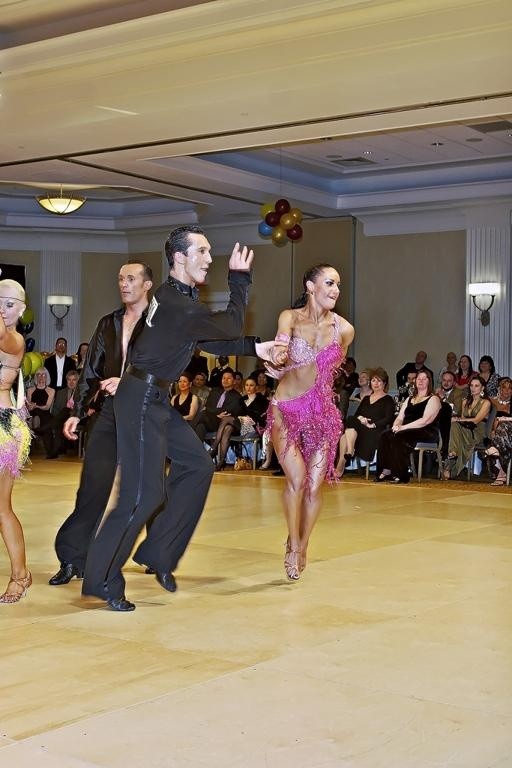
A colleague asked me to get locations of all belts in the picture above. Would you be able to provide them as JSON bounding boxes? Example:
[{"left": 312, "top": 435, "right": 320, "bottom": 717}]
[{"left": 125, "top": 363, "right": 172, "bottom": 390}]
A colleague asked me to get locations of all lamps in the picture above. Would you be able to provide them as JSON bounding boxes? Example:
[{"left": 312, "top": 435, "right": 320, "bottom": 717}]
[
  {"left": 47, "top": 296, "right": 74, "bottom": 330},
  {"left": 34, "top": 183, "right": 87, "bottom": 215}
]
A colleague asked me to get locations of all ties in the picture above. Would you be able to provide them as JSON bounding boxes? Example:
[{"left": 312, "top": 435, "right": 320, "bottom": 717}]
[{"left": 216, "top": 390, "right": 227, "bottom": 409}]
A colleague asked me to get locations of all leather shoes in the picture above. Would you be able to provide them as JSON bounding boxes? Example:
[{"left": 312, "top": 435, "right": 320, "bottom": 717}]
[
  {"left": 49, "top": 560, "right": 85, "bottom": 585},
  {"left": 83, "top": 588, "right": 136, "bottom": 611},
  {"left": 133, "top": 550, "right": 178, "bottom": 593}
]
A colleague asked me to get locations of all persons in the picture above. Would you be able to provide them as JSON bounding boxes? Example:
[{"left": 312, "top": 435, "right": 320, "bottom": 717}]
[
  {"left": 75, "top": 223, "right": 287, "bottom": 613},
  {"left": 0, "top": 277, "right": 35, "bottom": 605},
  {"left": 47, "top": 259, "right": 168, "bottom": 586},
  {"left": 170, "top": 345, "right": 285, "bottom": 476},
  {"left": 332, "top": 351, "right": 512, "bottom": 486},
  {"left": 257, "top": 263, "right": 358, "bottom": 581},
  {"left": 25, "top": 336, "right": 104, "bottom": 459}
]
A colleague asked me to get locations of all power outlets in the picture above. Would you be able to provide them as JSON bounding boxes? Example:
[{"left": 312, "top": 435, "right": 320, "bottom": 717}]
[{"left": 467, "top": 281, "right": 502, "bottom": 326}]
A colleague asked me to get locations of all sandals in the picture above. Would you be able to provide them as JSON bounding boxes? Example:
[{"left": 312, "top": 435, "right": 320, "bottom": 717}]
[
  {"left": 284, "top": 536, "right": 307, "bottom": 580},
  {"left": 480, "top": 448, "right": 499, "bottom": 460},
  {"left": 374, "top": 469, "right": 410, "bottom": 485},
  {"left": 491, "top": 474, "right": 507, "bottom": 487},
  {"left": 259, "top": 462, "right": 272, "bottom": 471}
]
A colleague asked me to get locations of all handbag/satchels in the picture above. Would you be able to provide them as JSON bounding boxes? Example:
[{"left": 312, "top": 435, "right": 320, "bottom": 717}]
[{"left": 233, "top": 442, "right": 253, "bottom": 470}]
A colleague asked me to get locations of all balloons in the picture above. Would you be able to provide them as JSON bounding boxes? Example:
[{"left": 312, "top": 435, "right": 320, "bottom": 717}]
[
  {"left": 257, "top": 196, "right": 303, "bottom": 247},
  {"left": 21, "top": 353, "right": 32, "bottom": 378}
]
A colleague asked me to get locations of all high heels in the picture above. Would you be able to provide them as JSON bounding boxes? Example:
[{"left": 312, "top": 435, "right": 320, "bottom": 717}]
[
  {"left": 215, "top": 459, "right": 227, "bottom": 472},
  {"left": 0, "top": 569, "right": 32, "bottom": 605}
]
